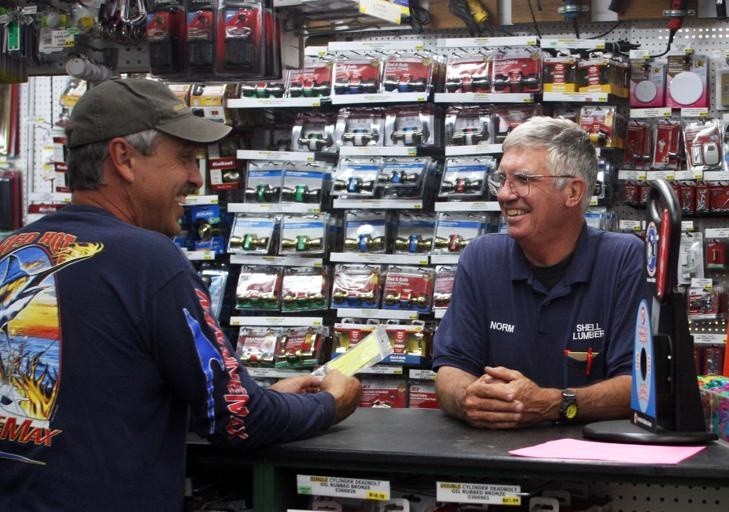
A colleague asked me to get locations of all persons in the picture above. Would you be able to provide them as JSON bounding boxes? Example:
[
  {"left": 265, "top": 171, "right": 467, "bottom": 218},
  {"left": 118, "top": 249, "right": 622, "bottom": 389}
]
[
  {"left": 0, "top": 76, "right": 362, "bottom": 512},
  {"left": 432, "top": 115, "right": 651, "bottom": 431}
]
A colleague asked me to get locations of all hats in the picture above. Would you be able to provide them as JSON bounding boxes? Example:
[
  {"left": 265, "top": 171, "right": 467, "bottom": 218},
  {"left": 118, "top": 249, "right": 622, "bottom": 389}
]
[{"left": 66, "top": 79, "right": 233, "bottom": 149}]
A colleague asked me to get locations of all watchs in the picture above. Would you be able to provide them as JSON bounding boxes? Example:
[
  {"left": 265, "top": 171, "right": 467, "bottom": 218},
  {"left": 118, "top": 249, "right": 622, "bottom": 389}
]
[{"left": 552, "top": 390, "right": 579, "bottom": 426}]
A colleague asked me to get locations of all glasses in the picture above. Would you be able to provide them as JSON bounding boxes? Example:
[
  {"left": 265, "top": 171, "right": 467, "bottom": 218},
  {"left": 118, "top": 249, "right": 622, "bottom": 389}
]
[{"left": 487, "top": 174, "right": 575, "bottom": 197}]
